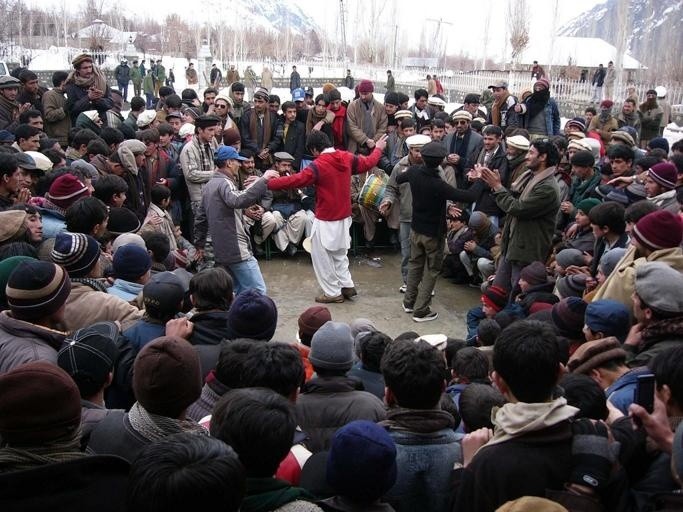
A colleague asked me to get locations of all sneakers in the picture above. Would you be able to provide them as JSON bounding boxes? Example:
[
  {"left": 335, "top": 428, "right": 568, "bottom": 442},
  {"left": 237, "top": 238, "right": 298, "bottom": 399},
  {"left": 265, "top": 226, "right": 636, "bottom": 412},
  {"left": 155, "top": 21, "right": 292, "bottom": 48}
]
[
  {"left": 315, "top": 294, "right": 344, "bottom": 304},
  {"left": 401, "top": 299, "right": 414, "bottom": 313},
  {"left": 341, "top": 286, "right": 357, "bottom": 298},
  {"left": 412, "top": 310, "right": 439, "bottom": 322},
  {"left": 399, "top": 282, "right": 407, "bottom": 293}
]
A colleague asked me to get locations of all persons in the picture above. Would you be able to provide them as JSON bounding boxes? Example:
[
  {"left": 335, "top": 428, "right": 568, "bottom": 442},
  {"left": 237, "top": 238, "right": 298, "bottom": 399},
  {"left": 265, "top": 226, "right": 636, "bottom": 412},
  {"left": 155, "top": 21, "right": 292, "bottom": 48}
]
[{"left": 1, "top": 54, "right": 682, "bottom": 512}]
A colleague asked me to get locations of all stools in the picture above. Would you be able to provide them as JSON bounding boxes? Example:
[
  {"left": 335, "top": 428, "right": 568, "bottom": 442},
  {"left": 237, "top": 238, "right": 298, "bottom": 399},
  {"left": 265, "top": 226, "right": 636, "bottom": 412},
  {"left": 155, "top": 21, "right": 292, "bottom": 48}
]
[
  {"left": 349, "top": 216, "right": 401, "bottom": 254},
  {"left": 263, "top": 228, "right": 311, "bottom": 263}
]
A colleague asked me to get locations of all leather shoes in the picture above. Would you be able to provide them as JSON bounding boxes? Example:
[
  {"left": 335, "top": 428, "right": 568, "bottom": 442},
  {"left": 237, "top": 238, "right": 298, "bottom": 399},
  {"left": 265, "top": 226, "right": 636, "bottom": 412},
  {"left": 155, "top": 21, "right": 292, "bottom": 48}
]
[{"left": 287, "top": 241, "right": 298, "bottom": 256}]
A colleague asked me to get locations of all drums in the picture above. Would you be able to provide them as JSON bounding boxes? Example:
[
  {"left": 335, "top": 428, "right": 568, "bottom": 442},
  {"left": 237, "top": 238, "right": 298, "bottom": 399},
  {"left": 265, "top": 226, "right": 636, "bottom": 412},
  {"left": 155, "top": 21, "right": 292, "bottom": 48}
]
[{"left": 358, "top": 174, "right": 393, "bottom": 216}]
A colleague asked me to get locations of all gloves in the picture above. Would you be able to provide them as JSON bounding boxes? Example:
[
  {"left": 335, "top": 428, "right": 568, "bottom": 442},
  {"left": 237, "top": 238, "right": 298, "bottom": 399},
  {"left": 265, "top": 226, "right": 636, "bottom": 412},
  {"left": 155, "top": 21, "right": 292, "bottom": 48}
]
[{"left": 569, "top": 416, "right": 619, "bottom": 489}]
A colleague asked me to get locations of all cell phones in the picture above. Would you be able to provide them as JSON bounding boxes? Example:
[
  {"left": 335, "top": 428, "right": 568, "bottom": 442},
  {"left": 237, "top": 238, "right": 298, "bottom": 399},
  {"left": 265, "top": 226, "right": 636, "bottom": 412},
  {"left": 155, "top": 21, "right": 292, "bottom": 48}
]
[{"left": 636, "top": 373, "right": 656, "bottom": 415}]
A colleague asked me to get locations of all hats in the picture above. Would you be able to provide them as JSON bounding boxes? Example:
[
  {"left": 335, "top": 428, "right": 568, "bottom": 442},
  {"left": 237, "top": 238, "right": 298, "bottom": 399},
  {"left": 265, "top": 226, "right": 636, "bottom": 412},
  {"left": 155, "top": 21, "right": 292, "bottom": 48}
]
[
  {"left": 325, "top": 421, "right": 398, "bottom": 500},
  {"left": 273, "top": 150, "right": 295, "bottom": 164},
  {"left": 71, "top": 53, "right": 92, "bottom": 67},
  {"left": 117, "top": 146, "right": 138, "bottom": 176},
  {"left": 566, "top": 211, "right": 683, "bottom": 375},
  {"left": 82, "top": 110, "right": 99, "bottom": 121},
  {"left": 599, "top": 100, "right": 612, "bottom": 107},
  {"left": 107, "top": 207, "right": 140, "bottom": 233},
  {"left": 308, "top": 318, "right": 356, "bottom": 370},
  {"left": 118, "top": 138, "right": 147, "bottom": 154},
  {"left": 0, "top": 232, "right": 184, "bottom": 319},
  {"left": 0, "top": 75, "right": 23, "bottom": 89},
  {"left": 488, "top": 79, "right": 585, "bottom": 131},
  {"left": 210, "top": 146, "right": 248, "bottom": 163},
  {"left": 481, "top": 247, "right": 587, "bottom": 339},
  {"left": 468, "top": 212, "right": 480, "bottom": 230},
  {"left": 0, "top": 151, "right": 99, "bottom": 243},
  {"left": 226, "top": 289, "right": 277, "bottom": 341},
  {"left": 298, "top": 304, "right": 334, "bottom": 334},
  {"left": 0, "top": 321, "right": 202, "bottom": 445},
  {"left": 413, "top": 334, "right": 447, "bottom": 351},
  {"left": 567, "top": 126, "right": 668, "bottom": 159},
  {"left": 577, "top": 162, "right": 677, "bottom": 211},
  {"left": 254, "top": 87, "right": 269, "bottom": 102},
  {"left": 291, "top": 82, "right": 341, "bottom": 103},
  {"left": 213, "top": 94, "right": 233, "bottom": 112},
  {"left": 356, "top": 80, "right": 449, "bottom": 159},
  {"left": 452, "top": 110, "right": 530, "bottom": 151},
  {"left": 135, "top": 107, "right": 221, "bottom": 138}
]
